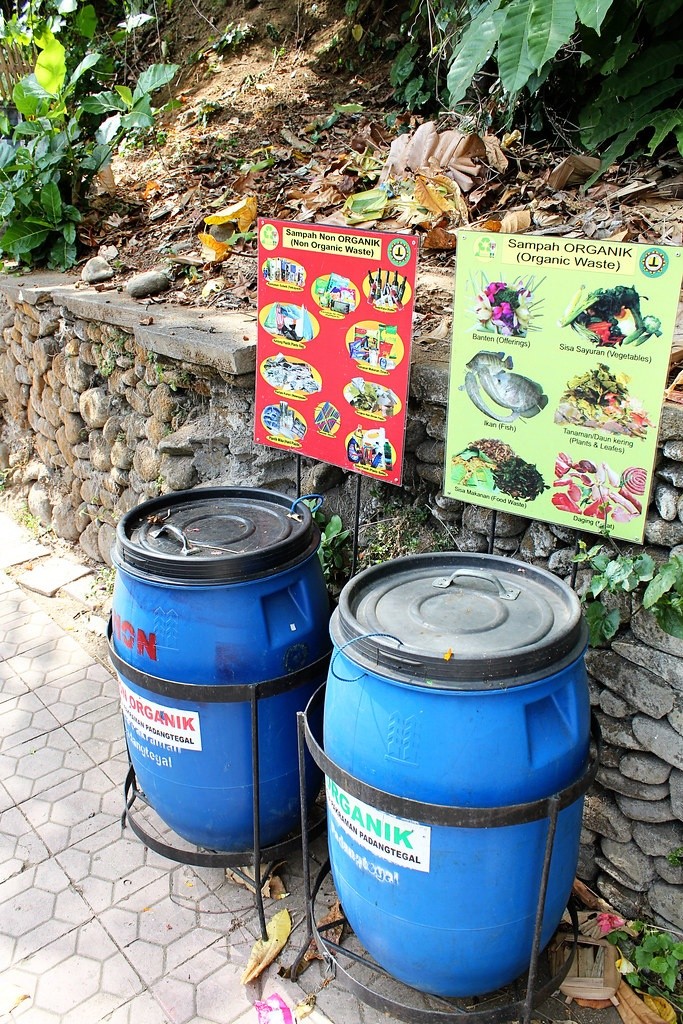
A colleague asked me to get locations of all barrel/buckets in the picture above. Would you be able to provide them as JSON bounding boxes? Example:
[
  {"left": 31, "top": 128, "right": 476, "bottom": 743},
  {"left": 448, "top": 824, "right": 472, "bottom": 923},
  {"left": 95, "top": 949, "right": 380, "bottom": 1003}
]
[
  {"left": 108, "top": 486, "right": 332, "bottom": 852},
  {"left": 324, "top": 551, "right": 590, "bottom": 999}
]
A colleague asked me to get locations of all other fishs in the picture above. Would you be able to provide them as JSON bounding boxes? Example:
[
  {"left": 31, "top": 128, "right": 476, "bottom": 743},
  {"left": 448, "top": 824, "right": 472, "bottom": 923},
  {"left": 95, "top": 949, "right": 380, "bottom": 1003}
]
[{"left": 459, "top": 349, "right": 549, "bottom": 425}]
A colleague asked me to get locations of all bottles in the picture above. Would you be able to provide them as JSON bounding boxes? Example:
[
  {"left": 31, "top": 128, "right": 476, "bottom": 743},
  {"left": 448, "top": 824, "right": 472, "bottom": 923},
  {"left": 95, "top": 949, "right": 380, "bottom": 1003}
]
[{"left": 367, "top": 268, "right": 407, "bottom": 305}]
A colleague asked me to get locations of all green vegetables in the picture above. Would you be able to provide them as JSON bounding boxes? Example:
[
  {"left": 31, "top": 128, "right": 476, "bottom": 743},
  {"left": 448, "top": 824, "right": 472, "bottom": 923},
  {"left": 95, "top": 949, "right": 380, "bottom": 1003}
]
[{"left": 560, "top": 286, "right": 659, "bottom": 347}]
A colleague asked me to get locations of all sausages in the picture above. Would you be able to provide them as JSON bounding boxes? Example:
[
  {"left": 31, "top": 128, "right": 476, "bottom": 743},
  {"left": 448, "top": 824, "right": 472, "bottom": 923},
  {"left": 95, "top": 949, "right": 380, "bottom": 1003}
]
[{"left": 622, "top": 467, "right": 647, "bottom": 495}]
